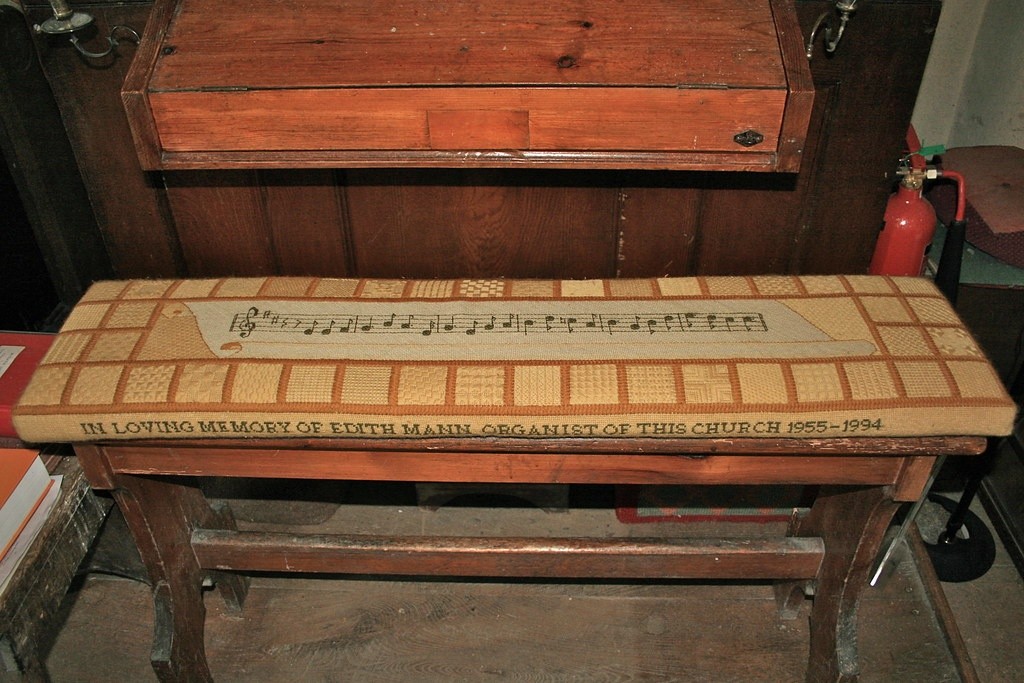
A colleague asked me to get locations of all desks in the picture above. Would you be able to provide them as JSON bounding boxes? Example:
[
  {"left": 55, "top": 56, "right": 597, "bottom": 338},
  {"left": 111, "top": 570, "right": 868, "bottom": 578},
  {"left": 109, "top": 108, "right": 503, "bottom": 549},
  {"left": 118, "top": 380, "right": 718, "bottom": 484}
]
[{"left": 13, "top": 274, "right": 1019, "bottom": 682}]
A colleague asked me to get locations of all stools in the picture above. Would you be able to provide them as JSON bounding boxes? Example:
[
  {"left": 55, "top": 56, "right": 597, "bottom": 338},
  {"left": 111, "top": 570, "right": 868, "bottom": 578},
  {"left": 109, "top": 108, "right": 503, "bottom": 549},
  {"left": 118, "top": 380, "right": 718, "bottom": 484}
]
[
  {"left": 898, "top": 212, "right": 1024, "bottom": 588},
  {"left": 0, "top": 328, "right": 208, "bottom": 683}
]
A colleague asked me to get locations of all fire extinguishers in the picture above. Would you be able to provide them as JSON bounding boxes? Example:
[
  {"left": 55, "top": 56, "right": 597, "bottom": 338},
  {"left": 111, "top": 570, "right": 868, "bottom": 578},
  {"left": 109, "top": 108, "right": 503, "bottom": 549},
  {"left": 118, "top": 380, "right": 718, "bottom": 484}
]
[{"left": 867, "top": 122, "right": 965, "bottom": 277}]
